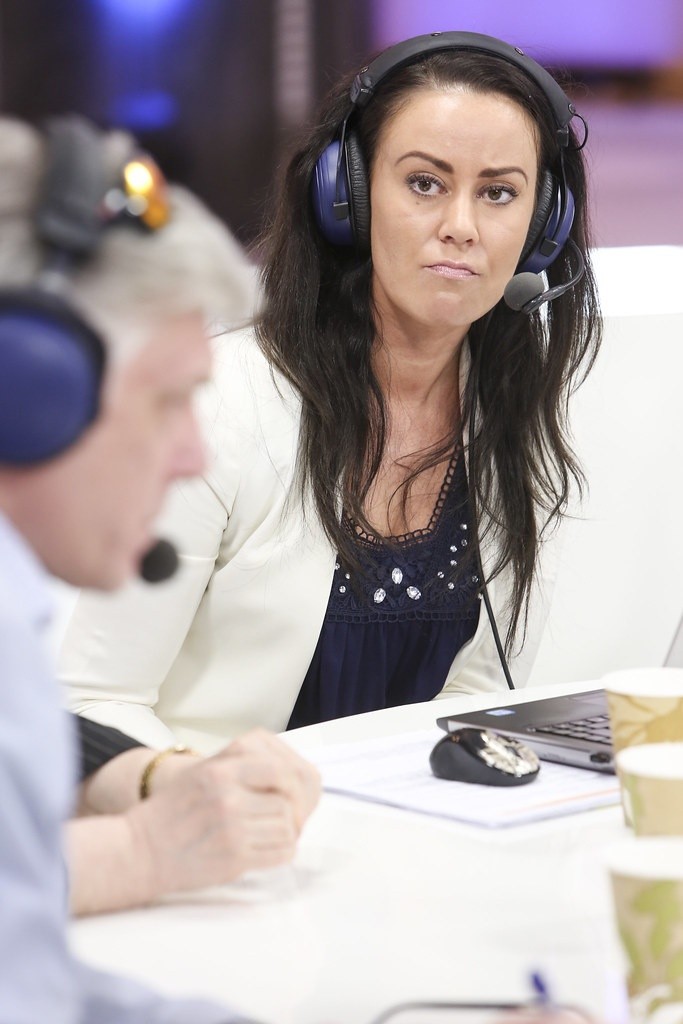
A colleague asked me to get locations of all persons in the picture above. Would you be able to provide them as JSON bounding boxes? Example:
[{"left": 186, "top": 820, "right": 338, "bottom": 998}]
[
  {"left": 64, "top": 34, "right": 603, "bottom": 755},
  {"left": 0, "top": 113, "right": 321, "bottom": 1024}
]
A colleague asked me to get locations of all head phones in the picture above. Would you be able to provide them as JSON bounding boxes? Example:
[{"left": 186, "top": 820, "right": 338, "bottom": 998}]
[
  {"left": 307, "top": 30, "right": 590, "bottom": 277},
  {"left": 1, "top": 115, "right": 113, "bottom": 469}
]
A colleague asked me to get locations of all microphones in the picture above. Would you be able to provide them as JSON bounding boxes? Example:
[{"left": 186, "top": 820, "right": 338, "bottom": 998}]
[
  {"left": 136, "top": 538, "right": 182, "bottom": 584},
  {"left": 504, "top": 244, "right": 584, "bottom": 316}
]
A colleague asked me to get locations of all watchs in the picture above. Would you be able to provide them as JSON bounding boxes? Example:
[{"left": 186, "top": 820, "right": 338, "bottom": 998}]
[{"left": 139, "top": 744, "right": 195, "bottom": 801}]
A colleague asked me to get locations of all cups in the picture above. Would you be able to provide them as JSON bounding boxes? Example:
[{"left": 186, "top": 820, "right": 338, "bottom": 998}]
[
  {"left": 612, "top": 740, "right": 683, "bottom": 843},
  {"left": 606, "top": 666, "right": 683, "bottom": 820},
  {"left": 611, "top": 848, "right": 682, "bottom": 1017}
]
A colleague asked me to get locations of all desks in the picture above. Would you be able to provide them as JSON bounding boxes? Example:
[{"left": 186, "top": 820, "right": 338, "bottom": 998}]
[{"left": 62, "top": 676, "right": 630, "bottom": 1024}]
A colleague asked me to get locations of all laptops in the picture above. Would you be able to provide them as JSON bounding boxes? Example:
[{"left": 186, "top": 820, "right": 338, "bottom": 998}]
[{"left": 435, "top": 617, "right": 683, "bottom": 776}]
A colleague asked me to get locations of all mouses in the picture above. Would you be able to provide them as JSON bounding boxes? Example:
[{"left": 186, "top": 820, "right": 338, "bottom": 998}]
[{"left": 428, "top": 728, "right": 541, "bottom": 787}]
